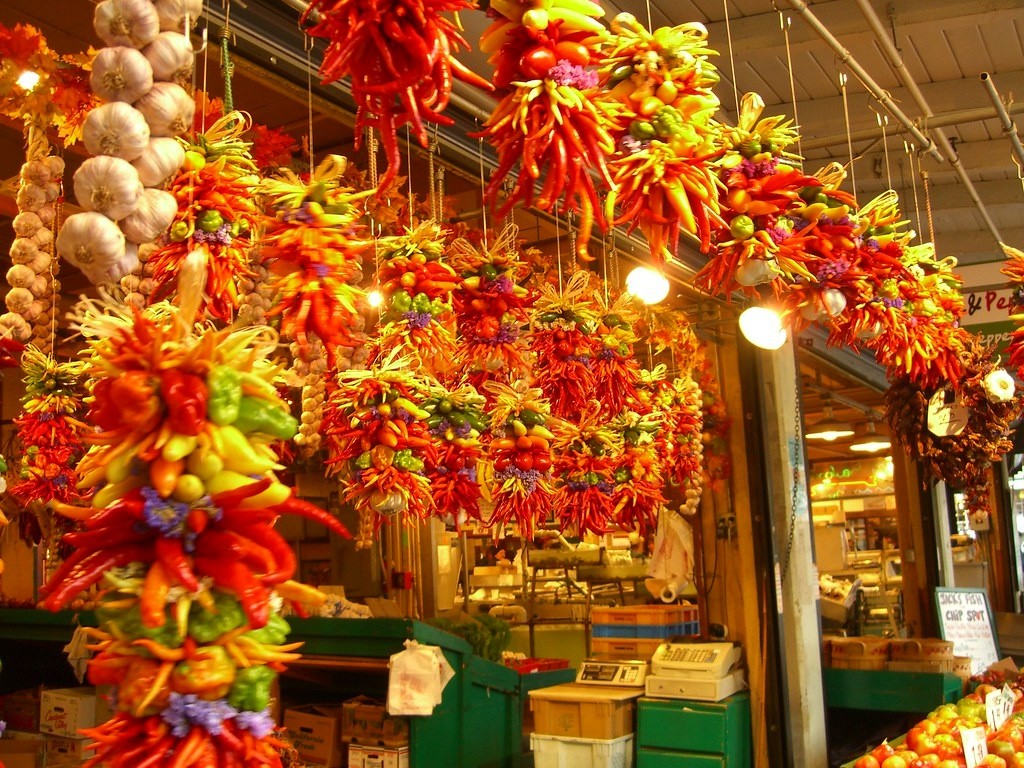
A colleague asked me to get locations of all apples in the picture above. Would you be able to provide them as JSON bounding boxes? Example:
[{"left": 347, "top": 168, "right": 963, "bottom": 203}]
[{"left": 850, "top": 687, "right": 1024, "bottom": 768}]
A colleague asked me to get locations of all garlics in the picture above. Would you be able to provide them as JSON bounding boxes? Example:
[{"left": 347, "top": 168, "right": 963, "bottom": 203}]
[{"left": 0, "top": 0, "right": 202, "bottom": 355}]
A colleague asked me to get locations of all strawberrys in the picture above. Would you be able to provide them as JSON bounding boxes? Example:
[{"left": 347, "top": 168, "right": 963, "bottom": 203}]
[{"left": 969, "top": 669, "right": 1024, "bottom": 694}]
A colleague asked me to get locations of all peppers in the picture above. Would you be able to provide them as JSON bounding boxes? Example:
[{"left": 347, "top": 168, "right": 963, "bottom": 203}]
[
  {"left": 0, "top": 145, "right": 704, "bottom": 768},
  {"left": 299, "top": 0, "right": 1024, "bottom": 518}
]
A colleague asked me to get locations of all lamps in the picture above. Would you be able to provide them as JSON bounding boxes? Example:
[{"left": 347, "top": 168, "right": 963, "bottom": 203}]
[
  {"left": 849, "top": 411, "right": 891, "bottom": 453},
  {"left": 805, "top": 395, "right": 856, "bottom": 441}
]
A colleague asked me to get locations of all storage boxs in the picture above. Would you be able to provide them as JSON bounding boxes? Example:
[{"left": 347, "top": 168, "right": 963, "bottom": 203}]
[
  {"left": 341, "top": 694, "right": 408, "bottom": 748},
  {"left": 283, "top": 703, "right": 346, "bottom": 768},
  {"left": 529, "top": 684, "right": 644, "bottom": 739},
  {"left": 348, "top": 744, "right": 409, "bottom": 768},
  {"left": 278, "top": 467, "right": 382, "bottom": 598},
  {"left": 819, "top": 579, "right": 863, "bottom": 622},
  {"left": 505, "top": 658, "right": 571, "bottom": 674},
  {"left": 529, "top": 731, "right": 635, "bottom": 768},
  {"left": 591, "top": 604, "right": 699, "bottom": 654}
]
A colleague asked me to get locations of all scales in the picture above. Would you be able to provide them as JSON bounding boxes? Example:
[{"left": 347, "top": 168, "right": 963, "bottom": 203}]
[{"left": 575, "top": 655, "right": 651, "bottom": 688}]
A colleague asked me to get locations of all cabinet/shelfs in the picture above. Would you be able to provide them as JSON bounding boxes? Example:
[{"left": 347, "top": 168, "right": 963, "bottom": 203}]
[{"left": 633, "top": 693, "right": 751, "bottom": 768}]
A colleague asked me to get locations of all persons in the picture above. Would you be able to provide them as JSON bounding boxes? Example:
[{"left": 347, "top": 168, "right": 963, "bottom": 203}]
[{"left": 489, "top": 545, "right": 506, "bottom": 567}]
